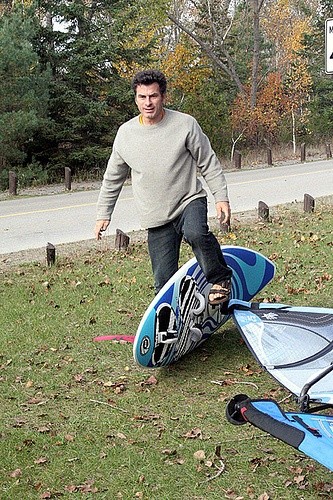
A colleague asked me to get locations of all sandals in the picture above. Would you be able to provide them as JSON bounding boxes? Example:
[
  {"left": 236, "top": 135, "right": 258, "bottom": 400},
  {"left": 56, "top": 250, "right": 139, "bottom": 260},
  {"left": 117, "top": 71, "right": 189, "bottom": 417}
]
[{"left": 208, "top": 278, "right": 232, "bottom": 303}]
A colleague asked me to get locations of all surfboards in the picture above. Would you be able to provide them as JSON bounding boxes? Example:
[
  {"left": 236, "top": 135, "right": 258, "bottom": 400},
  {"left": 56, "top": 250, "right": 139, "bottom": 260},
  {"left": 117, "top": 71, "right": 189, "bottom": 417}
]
[{"left": 131, "top": 246, "right": 277, "bottom": 368}]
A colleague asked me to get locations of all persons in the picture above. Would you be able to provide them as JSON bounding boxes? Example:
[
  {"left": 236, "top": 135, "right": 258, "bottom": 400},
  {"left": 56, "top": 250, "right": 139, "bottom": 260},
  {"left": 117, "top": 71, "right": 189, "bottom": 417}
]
[{"left": 94, "top": 70, "right": 232, "bottom": 305}]
